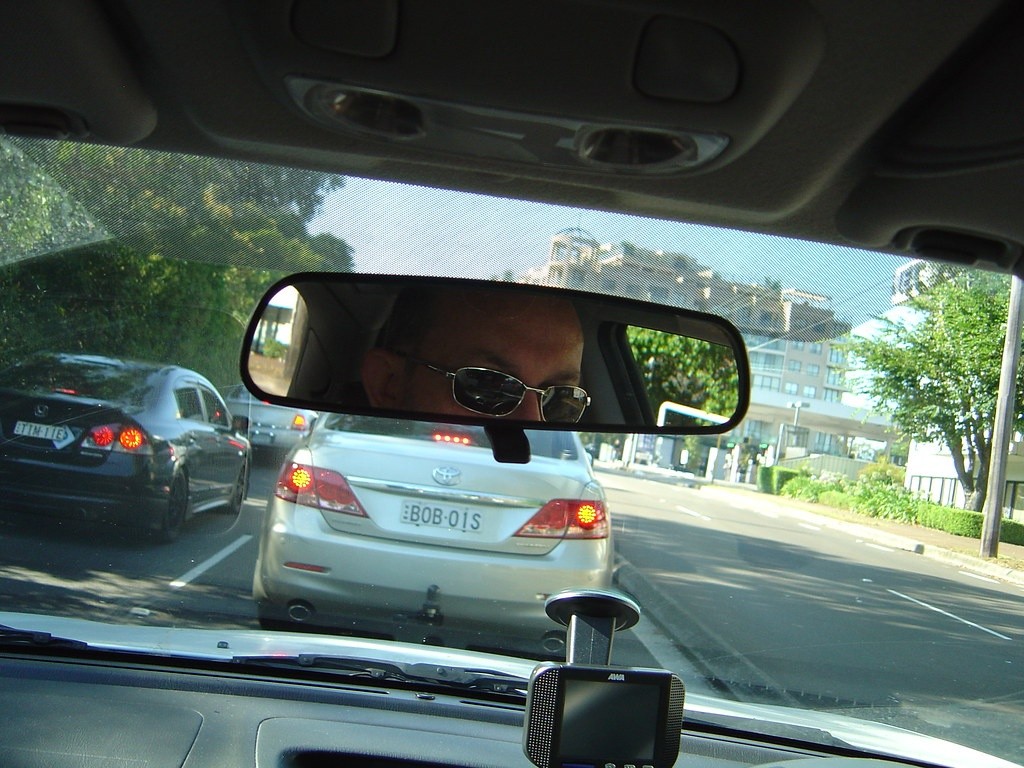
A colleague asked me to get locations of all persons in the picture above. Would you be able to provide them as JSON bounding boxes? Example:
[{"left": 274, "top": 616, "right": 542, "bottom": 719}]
[
  {"left": 724, "top": 448, "right": 734, "bottom": 481},
  {"left": 610, "top": 438, "right": 622, "bottom": 471},
  {"left": 360, "top": 281, "right": 591, "bottom": 424}
]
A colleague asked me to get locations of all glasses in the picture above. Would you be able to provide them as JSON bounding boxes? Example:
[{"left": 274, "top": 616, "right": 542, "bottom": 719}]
[{"left": 390, "top": 349, "right": 591, "bottom": 422}]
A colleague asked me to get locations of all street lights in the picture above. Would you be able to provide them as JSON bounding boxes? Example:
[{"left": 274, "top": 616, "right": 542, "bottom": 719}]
[{"left": 788, "top": 401, "right": 811, "bottom": 427}]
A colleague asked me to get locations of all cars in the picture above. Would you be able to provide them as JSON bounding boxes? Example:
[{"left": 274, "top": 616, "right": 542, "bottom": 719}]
[
  {"left": 251, "top": 408, "right": 617, "bottom": 670},
  {"left": 225, "top": 383, "right": 319, "bottom": 448},
  {"left": 0, "top": 351, "right": 253, "bottom": 544}
]
